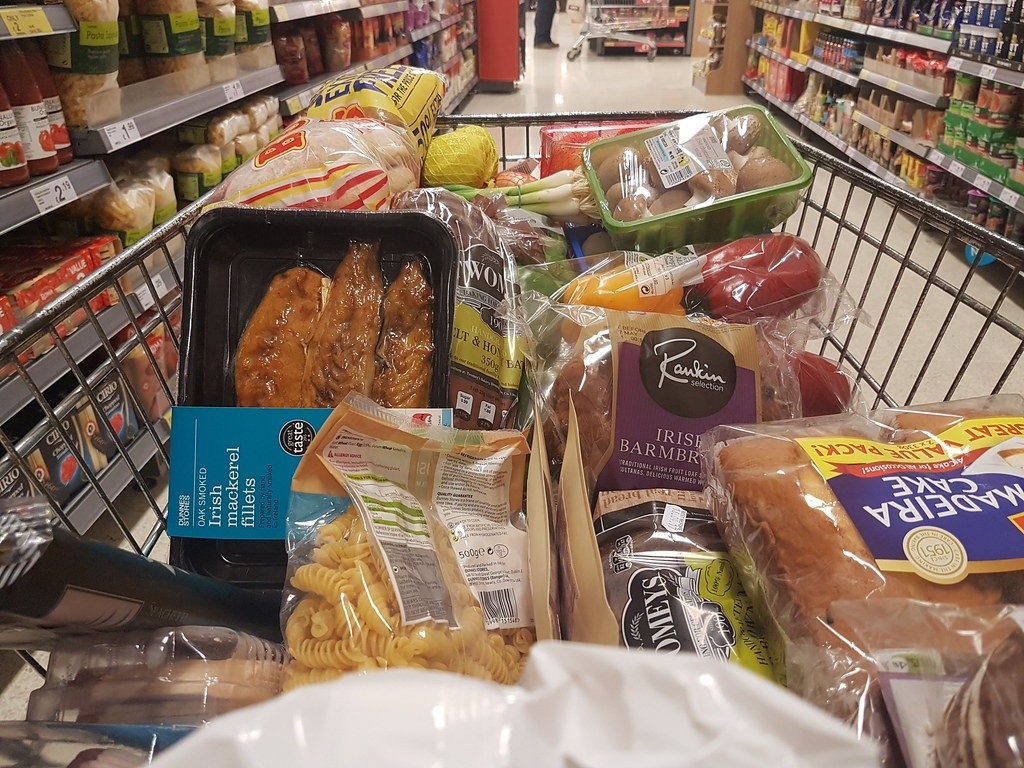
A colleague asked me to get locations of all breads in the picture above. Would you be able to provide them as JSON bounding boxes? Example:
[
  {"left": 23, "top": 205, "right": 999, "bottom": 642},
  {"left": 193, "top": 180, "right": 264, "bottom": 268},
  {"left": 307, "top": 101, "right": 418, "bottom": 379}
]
[{"left": 717, "top": 406, "right": 1024, "bottom": 724}]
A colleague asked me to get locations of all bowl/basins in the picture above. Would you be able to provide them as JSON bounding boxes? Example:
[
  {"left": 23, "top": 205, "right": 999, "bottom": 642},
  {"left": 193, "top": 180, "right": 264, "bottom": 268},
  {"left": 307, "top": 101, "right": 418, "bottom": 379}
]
[{"left": 581, "top": 104, "right": 814, "bottom": 256}]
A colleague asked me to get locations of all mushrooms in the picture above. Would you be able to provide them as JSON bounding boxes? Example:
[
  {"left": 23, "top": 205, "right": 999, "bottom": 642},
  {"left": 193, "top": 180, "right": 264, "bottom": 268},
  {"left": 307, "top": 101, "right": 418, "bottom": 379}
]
[{"left": 594, "top": 113, "right": 793, "bottom": 223}]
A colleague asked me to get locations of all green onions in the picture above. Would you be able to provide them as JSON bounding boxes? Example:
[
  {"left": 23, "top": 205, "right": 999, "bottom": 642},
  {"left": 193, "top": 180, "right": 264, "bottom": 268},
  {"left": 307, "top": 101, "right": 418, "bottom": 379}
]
[{"left": 445, "top": 165, "right": 596, "bottom": 218}]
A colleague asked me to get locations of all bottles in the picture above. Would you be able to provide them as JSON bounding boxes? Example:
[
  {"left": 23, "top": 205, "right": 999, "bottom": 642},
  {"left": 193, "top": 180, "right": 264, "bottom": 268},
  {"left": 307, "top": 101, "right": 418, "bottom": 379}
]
[{"left": 0, "top": 36, "right": 75, "bottom": 188}]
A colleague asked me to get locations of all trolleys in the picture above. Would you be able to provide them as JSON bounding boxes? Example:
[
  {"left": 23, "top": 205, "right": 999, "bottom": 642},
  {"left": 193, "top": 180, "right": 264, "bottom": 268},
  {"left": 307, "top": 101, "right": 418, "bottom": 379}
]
[{"left": 568, "top": 0, "right": 669, "bottom": 62}]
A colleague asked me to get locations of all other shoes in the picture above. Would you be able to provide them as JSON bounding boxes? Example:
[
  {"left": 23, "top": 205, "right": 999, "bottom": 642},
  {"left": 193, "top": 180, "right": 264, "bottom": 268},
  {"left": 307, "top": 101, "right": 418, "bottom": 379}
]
[
  {"left": 534, "top": 41, "right": 553, "bottom": 50},
  {"left": 549, "top": 42, "right": 560, "bottom": 47}
]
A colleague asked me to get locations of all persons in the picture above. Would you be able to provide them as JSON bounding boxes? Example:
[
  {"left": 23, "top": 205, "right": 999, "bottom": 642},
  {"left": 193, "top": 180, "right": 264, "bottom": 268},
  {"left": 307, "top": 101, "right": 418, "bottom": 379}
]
[{"left": 532, "top": 0, "right": 560, "bottom": 49}]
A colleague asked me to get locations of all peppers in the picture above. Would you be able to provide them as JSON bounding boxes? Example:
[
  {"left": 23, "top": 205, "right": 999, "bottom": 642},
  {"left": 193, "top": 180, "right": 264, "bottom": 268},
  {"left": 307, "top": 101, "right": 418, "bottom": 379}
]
[{"left": 559, "top": 232, "right": 853, "bottom": 424}]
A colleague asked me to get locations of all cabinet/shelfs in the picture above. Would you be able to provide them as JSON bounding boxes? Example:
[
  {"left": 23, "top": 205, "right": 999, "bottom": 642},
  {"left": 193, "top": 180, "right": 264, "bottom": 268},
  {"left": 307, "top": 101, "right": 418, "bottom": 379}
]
[
  {"left": 586, "top": 0, "right": 695, "bottom": 57},
  {"left": 690, "top": 0, "right": 749, "bottom": 94},
  {"left": 741, "top": 0, "right": 1024, "bottom": 277},
  {"left": 0, "top": 0, "right": 478, "bottom": 591}
]
